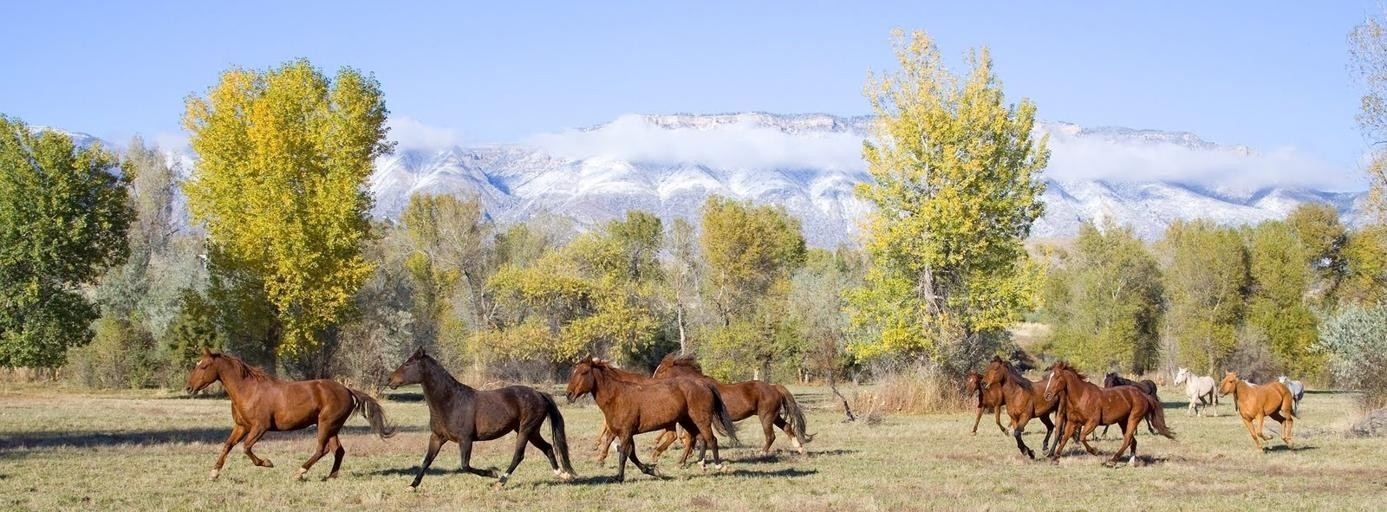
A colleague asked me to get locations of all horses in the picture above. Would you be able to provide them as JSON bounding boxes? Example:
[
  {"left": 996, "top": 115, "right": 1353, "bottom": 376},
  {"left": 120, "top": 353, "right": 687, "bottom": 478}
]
[
  {"left": 1174, "top": 366, "right": 1218, "bottom": 419},
  {"left": 184, "top": 346, "right": 402, "bottom": 483},
  {"left": 966, "top": 354, "right": 1179, "bottom": 469},
  {"left": 565, "top": 351, "right": 817, "bottom": 483},
  {"left": 387, "top": 345, "right": 577, "bottom": 493},
  {"left": 1218, "top": 369, "right": 1304, "bottom": 453}
]
